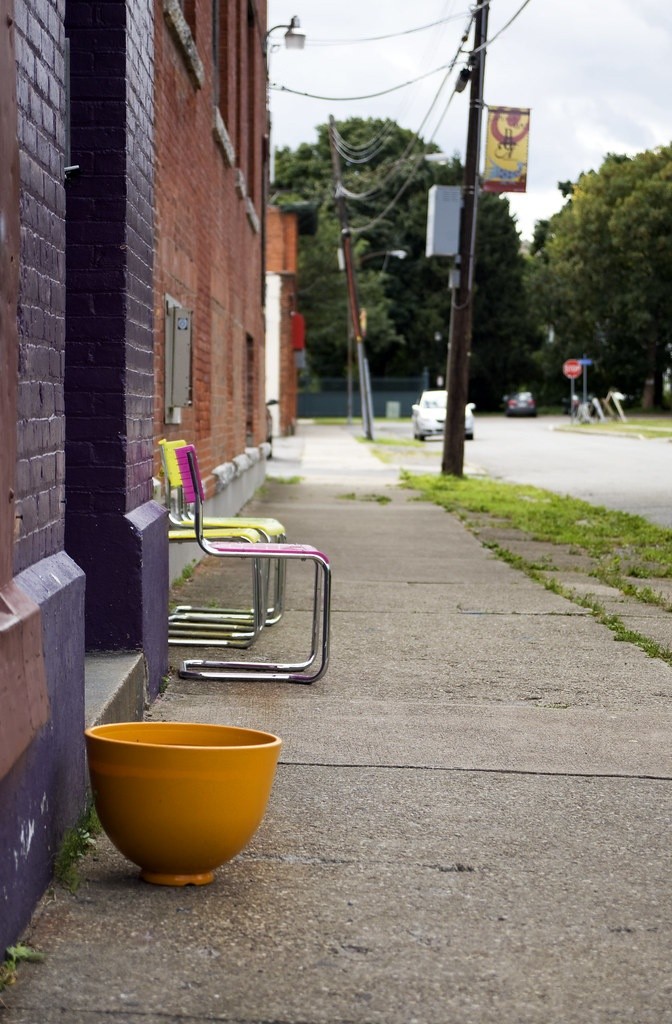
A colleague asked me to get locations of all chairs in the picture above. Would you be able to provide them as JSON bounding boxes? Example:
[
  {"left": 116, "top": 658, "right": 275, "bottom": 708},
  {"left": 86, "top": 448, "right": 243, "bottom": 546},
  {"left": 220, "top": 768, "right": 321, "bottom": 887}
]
[{"left": 157, "top": 437, "right": 332, "bottom": 683}]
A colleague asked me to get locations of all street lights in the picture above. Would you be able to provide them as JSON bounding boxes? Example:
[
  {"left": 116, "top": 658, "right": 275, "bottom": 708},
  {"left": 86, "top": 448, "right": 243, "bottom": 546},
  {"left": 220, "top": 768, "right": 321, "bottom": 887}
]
[{"left": 344, "top": 247, "right": 408, "bottom": 423}]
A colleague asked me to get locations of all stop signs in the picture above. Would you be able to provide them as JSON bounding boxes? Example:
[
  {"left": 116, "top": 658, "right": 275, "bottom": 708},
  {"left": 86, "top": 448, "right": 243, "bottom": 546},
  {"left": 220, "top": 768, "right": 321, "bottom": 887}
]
[{"left": 562, "top": 358, "right": 582, "bottom": 379}]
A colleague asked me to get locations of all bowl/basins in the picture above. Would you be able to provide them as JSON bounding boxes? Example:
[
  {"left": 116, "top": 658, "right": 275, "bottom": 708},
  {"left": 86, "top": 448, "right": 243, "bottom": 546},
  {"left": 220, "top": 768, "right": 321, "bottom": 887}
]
[{"left": 82, "top": 720, "right": 283, "bottom": 886}]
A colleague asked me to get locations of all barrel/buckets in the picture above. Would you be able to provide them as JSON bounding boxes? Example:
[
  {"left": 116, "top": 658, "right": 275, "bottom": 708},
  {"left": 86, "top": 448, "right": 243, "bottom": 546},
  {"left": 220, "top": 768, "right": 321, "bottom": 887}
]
[{"left": 385, "top": 401, "right": 400, "bottom": 418}]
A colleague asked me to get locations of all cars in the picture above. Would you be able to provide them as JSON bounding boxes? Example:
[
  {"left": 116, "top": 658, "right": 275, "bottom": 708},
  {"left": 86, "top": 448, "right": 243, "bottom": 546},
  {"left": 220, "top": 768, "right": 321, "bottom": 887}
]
[
  {"left": 502, "top": 391, "right": 538, "bottom": 417},
  {"left": 410, "top": 389, "right": 477, "bottom": 440},
  {"left": 560, "top": 390, "right": 596, "bottom": 415}
]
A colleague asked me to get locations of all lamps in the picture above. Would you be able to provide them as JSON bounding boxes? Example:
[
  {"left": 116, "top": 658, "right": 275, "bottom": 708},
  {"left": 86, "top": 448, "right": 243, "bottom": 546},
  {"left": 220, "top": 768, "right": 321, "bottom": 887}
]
[{"left": 263, "top": 17, "right": 307, "bottom": 57}]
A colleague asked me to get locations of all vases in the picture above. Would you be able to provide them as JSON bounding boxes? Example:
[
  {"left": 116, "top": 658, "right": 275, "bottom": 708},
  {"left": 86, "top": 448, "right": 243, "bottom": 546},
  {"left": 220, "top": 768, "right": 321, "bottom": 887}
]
[{"left": 84, "top": 722, "right": 283, "bottom": 885}]
